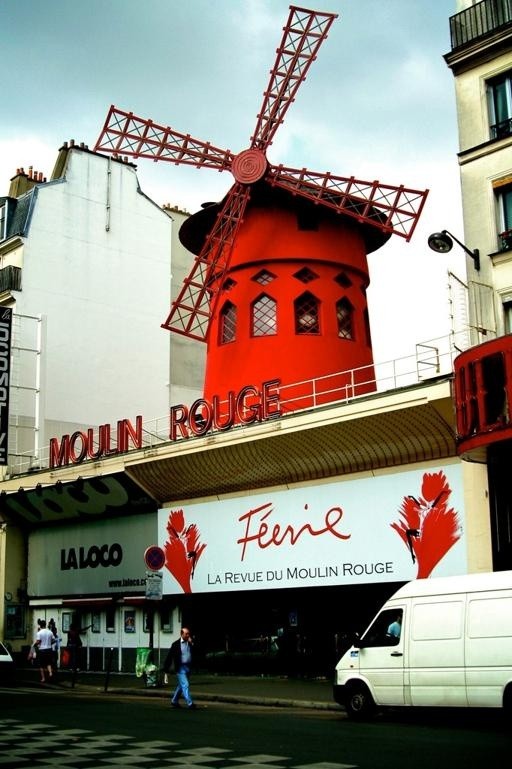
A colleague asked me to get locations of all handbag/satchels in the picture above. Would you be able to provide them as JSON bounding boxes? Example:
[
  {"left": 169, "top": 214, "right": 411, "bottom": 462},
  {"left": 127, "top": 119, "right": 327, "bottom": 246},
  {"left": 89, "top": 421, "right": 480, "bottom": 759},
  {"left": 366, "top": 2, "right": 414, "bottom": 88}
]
[
  {"left": 28, "top": 647, "right": 36, "bottom": 661},
  {"left": 62, "top": 650, "right": 69, "bottom": 665}
]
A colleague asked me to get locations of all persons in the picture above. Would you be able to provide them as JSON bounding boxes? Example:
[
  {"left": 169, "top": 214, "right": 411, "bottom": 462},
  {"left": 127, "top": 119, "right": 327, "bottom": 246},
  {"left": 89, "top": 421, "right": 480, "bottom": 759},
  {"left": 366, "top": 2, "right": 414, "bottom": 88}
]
[
  {"left": 31, "top": 619, "right": 82, "bottom": 683},
  {"left": 160, "top": 627, "right": 199, "bottom": 710},
  {"left": 385, "top": 611, "right": 401, "bottom": 641}
]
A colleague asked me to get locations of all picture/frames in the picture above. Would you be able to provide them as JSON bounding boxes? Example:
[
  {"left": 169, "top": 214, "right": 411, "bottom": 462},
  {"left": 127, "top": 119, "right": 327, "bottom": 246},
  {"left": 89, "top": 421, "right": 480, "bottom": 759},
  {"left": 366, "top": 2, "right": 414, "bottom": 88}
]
[{"left": 62, "top": 608, "right": 174, "bottom": 633}]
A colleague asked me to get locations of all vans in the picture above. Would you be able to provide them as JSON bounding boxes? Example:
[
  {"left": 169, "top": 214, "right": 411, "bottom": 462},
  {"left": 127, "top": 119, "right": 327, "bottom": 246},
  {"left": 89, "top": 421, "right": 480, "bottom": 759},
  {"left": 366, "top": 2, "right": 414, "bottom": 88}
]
[{"left": 330, "top": 569, "right": 511, "bottom": 726}]
